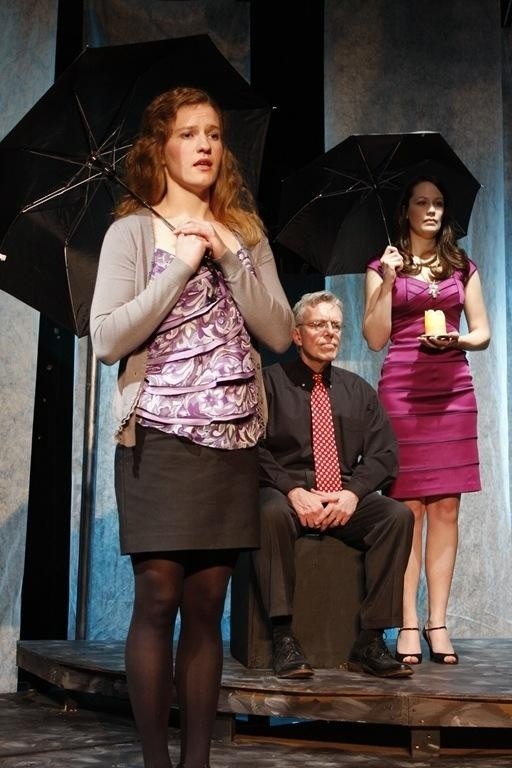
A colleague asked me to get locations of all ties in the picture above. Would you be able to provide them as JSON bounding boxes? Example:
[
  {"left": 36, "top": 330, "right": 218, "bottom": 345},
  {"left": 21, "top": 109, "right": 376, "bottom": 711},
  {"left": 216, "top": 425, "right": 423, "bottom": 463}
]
[{"left": 309, "top": 372, "right": 342, "bottom": 492}]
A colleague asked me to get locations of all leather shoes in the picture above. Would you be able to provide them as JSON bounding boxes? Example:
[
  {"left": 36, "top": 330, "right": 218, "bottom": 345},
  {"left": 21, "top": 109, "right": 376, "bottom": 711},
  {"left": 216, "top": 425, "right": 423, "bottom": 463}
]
[
  {"left": 349, "top": 634, "right": 414, "bottom": 680},
  {"left": 273, "top": 635, "right": 314, "bottom": 680}
]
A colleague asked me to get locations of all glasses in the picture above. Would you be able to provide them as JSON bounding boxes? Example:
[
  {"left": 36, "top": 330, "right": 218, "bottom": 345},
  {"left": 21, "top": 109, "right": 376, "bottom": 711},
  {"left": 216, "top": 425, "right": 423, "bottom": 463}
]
[{"left": 296, "top": 320, "right": 342, "bottom": 334}]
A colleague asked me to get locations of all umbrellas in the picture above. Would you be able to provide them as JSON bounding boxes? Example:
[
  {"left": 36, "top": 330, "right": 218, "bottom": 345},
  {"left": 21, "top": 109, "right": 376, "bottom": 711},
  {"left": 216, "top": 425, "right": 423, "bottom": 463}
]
[
  {"left": 255, "top": 126, "right": 486, "bottom": 279},
  {"left": 0, "top": 30, "right": 284, "bottom": 348}
]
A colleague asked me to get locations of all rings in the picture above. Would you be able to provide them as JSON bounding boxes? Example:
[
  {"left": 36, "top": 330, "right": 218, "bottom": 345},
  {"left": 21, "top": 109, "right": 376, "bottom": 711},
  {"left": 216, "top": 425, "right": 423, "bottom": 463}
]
[{"left": 334, "top": 518, "right": 340, "bottom": 523}]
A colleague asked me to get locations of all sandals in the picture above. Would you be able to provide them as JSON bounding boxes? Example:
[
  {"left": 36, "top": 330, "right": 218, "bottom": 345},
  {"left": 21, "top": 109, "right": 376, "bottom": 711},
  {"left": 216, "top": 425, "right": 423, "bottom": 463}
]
[{"left": 395, "top": 626, "right": 458, "bottom": 665}]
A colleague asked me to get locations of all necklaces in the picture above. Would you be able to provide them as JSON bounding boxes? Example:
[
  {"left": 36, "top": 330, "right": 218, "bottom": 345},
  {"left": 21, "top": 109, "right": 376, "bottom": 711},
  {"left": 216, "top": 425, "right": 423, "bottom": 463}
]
[{"left": 411, "top": 252, "right": 441, "bottom": 299}]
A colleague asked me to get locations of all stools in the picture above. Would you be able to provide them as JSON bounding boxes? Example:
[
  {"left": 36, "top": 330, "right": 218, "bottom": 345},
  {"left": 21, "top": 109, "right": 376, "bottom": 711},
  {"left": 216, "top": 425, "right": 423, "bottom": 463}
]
[{"left": 231, "top": 534, "right": 362, "bottom": 675}]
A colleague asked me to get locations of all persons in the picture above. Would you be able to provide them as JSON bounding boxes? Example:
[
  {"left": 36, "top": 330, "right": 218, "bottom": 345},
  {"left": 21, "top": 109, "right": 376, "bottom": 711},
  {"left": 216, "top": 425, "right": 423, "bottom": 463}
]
[
  {"left": 88, "top": 84, "right": 300, "bottom": 768},
  {"left": 360, "top": 175, "right": 492, "bottom": 667},
  {"left": 243, "top": 291, "right": 418, "bottom": 681}
]
